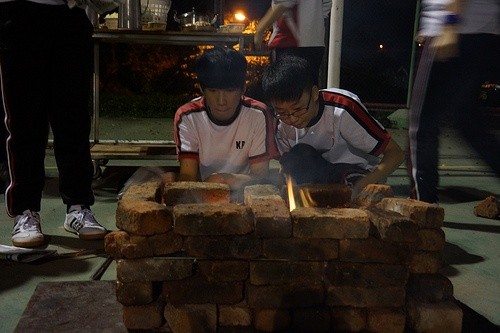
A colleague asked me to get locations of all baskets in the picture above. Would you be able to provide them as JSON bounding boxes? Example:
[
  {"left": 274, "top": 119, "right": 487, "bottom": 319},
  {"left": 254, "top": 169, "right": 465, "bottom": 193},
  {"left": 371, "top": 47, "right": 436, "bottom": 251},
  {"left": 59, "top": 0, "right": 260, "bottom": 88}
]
[
  {"left": 140, "top": 0, "right": 171, "bottom": 30},
  {"left": 104, "top": 17, "right": 118, "bottom": 29}
]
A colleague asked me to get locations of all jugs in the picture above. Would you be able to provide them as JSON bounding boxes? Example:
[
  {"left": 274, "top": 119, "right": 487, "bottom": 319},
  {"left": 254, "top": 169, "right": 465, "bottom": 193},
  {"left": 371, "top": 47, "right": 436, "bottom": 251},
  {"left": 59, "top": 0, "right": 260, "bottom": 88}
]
[{"left": 118, "top": 0, "right": 149, "bottom": 30}]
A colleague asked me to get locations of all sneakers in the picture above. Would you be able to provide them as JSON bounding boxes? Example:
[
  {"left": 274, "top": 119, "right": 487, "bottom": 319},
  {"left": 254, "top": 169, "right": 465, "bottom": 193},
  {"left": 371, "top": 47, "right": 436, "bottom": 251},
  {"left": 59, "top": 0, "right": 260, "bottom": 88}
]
[
  {"left": 63, "top": 208, "right": 108, "bottom": 240},
  {"left": 11, "top": 209, "right": 45, "bottom": 247}
]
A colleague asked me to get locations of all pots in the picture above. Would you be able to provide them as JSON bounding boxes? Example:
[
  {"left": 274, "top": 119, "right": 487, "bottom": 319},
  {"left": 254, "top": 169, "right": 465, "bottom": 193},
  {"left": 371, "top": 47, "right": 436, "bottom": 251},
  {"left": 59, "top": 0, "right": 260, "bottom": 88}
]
[{"left": 172, "top": 7, "right": 220, "bottom": 31}]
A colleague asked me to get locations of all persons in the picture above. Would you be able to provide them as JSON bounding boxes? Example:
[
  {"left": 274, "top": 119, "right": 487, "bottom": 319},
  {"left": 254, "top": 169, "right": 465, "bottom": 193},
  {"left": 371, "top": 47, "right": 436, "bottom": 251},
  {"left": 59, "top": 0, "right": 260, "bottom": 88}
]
[
  {"left": 0, "top": 0, "right": 108, "bottom": 247},
  {"left": 165, "top": 0, "right": 216, "bottom": 32},
  {"left": 254, "top": 0, "right": 331, "bottom": 88},
  {"left": 174, "top": 47, "right": 276, "bottom": 190},
  {"left": 406, "top": 0, "right": 500, "bottom": 217},
  {"left": 261, "top": 54, "right": 405, "bottom": 204}
]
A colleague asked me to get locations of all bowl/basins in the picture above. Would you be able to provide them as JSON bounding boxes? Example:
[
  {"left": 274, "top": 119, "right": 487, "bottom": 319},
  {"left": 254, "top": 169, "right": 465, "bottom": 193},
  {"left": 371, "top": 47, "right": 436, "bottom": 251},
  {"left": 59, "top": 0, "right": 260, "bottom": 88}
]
[
  {"left": 219, "top": 24, "right": 245, "bottom": 33},
  {"left": 103, "top": 18, "right": 118, "bottom": 29}
]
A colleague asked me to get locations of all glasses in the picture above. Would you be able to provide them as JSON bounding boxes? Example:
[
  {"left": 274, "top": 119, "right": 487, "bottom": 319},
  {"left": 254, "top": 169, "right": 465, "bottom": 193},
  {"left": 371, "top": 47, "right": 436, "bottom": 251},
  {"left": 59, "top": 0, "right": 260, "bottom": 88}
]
[{"left": 272, "top": 88, "right": 311, "bottom": 121}]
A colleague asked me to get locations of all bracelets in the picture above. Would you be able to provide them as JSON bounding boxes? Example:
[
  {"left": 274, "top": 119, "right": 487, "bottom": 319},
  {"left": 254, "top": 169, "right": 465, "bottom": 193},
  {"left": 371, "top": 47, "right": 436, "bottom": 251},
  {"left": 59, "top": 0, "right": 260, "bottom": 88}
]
[{"left": 441, "top": 14, "right": 461, "bottom": 26}]
